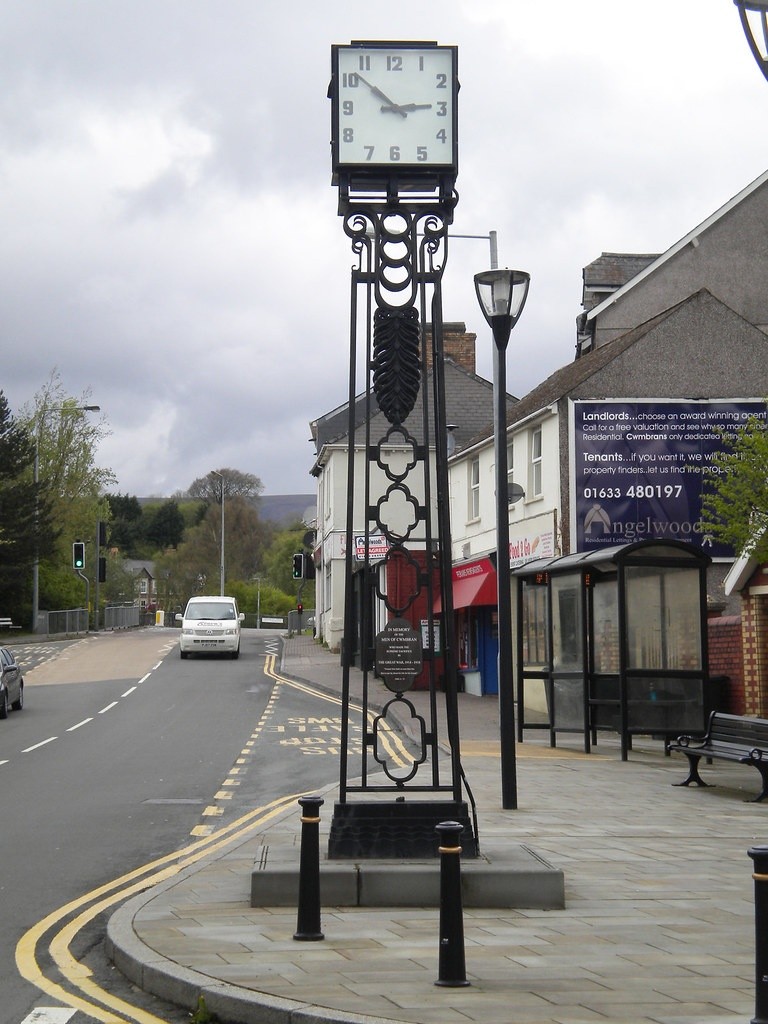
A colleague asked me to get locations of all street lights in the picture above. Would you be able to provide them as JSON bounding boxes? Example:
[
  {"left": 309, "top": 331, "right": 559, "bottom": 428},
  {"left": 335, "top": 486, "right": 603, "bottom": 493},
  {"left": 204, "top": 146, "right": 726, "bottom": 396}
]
[
  {"left": 253, "top": 577, "right": 260, "bottom": 629},
  {"left": 211, "top": 470, "right": 225, "bottom": 596},
  {"left": 31, "top": 405, "right": 102, "bottom": 635},
  {"left": 472, "top": 267, "right": 531, "bottom": 811}
]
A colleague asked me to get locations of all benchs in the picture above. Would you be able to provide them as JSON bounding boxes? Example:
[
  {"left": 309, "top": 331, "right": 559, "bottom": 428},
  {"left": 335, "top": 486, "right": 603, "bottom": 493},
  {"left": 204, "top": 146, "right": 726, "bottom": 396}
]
[{"left": 667, "top": 710, "right": 768, "bottom": 804}]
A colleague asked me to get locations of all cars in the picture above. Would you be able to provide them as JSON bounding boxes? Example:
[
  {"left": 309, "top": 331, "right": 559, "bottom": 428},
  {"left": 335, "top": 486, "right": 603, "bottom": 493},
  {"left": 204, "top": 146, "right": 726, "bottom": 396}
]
[{"left": 0, "top": 648, "right": 24, "bottom": 719}]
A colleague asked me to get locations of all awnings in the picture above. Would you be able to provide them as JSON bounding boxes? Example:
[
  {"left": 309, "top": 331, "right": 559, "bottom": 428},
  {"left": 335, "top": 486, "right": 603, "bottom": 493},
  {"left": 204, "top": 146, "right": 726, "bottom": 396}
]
[{"left": 433, "top": 572, "right": 498, "bottom": 614}]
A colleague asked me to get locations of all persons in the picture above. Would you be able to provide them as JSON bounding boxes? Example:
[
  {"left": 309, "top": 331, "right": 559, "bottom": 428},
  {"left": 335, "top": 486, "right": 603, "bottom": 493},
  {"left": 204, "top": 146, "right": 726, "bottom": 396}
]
[{"left": 145, "top": 603, "right": 153, "bottom": 626}]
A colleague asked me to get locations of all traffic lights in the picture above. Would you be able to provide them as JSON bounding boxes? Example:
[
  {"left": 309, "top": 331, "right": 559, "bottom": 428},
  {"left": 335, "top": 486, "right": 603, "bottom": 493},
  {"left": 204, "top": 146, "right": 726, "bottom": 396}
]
[
  {"left": 297, "top": 604, "right": 303, "bottom": 615},
  {"left": 293, "top": 553, "right": 304, "bottom": 579},
  {"left": 73, "top": 542, "right": 86, "bottom": 570}
]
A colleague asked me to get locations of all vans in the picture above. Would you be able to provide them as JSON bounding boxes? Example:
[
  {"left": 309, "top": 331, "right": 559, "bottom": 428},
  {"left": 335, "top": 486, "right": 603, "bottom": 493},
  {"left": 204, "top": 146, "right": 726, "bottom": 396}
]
[{"left": 175, "top": 596, "right": 245, "bottom": 659}]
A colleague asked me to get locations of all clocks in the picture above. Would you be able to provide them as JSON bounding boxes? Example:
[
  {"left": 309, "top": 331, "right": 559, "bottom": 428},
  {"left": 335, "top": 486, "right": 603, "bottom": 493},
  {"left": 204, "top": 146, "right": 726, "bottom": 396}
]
[{"left": 333, "top": 46, "right": 456, "bottom": 167}]
